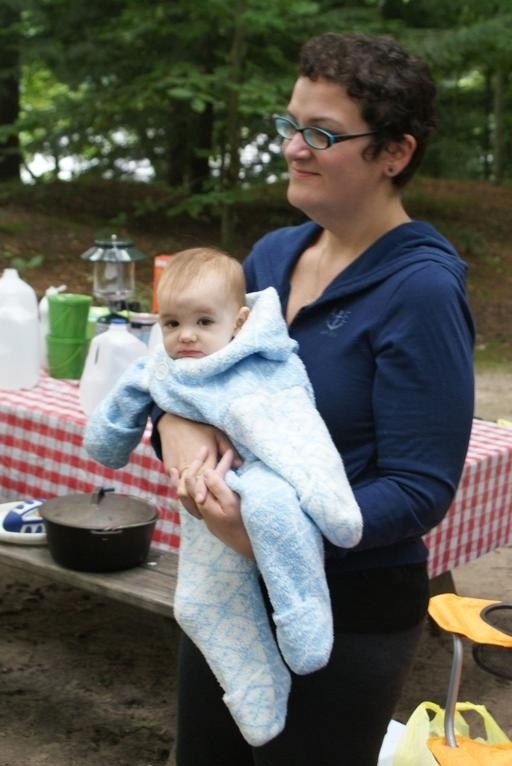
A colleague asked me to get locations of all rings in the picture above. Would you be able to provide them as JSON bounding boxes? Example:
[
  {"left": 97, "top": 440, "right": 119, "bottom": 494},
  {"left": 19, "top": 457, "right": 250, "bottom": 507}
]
[{"left": 178, "top": 466, "right": 191, "bottom": 479}]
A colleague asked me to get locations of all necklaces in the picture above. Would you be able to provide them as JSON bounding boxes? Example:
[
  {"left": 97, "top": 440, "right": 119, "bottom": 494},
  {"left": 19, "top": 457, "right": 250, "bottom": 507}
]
[{"left": 307, "top": 237, "right": 366, "bottom": 299}]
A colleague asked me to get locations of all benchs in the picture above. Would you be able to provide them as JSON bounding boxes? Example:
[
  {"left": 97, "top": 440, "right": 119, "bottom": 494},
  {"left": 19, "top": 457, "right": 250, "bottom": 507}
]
[{"left": 0, "top": 538, "right": 216, "bottom": 765}]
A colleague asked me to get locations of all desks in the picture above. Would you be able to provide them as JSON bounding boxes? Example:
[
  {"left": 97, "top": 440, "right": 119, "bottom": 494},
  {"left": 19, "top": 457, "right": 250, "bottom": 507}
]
[{"left": 0, "top": 368, "right": 512, "bottom": 638}]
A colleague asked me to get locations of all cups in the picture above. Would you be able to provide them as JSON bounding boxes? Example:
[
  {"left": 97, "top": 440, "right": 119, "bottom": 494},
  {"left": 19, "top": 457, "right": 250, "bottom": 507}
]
[{"left": 79, "top": 237, "right": 148, "bottom": 301}]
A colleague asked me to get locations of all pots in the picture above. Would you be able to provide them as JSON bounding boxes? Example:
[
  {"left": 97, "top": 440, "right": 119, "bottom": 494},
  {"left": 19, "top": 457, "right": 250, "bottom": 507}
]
[{"left": 39, "top": 485, "right": 160, "bottom": 574}]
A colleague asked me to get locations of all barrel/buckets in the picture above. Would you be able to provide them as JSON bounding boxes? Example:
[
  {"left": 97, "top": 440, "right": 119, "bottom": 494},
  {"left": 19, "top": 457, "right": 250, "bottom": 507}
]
[
  {"left": 80, "top": 318, "right": 149, "bottom": 417},
  {"left": 38, "top": 285, "right": 66, "bottom": 368}
]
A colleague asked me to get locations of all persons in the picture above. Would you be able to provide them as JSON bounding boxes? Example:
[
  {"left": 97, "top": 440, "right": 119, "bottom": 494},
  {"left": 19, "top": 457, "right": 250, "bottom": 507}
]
[
  {"left": 82, "top": 246, "right": 366, "bottom": 748},
  {"left": 170, "top": 27, "right": 475, "bottom": 764}
]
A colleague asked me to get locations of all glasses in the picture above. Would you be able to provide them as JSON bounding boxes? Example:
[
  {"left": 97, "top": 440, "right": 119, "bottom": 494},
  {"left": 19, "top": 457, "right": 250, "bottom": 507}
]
[{"left": 268, "top": 113, "right": 382, "bottom": 154}]
